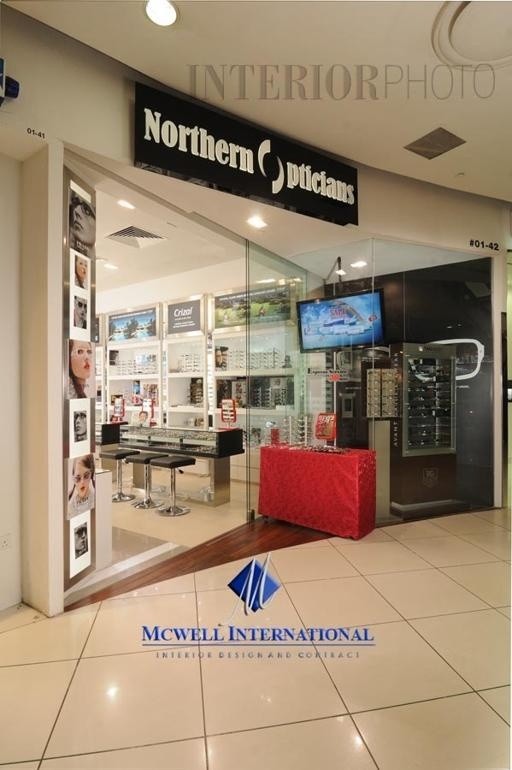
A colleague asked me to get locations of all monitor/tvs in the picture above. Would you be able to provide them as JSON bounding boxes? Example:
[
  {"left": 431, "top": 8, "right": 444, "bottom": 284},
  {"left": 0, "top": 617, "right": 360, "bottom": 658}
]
[{"left": 295, "top": 288, "right": 383, "bottom": 354}]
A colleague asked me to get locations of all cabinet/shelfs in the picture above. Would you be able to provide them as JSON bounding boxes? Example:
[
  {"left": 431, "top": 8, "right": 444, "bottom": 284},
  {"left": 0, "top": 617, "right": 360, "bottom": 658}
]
[{"left": 95, "top": 328, "right": 299, "bottom": 484}]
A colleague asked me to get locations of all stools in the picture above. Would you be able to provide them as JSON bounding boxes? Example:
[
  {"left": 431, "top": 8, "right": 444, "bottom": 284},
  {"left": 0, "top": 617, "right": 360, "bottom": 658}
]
[{"left": 99, "top": 448, "right": 196, "bottom": 518}]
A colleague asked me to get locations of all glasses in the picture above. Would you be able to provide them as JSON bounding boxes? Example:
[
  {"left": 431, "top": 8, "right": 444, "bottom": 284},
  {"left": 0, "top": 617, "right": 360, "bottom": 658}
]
[
  {"left": 73, "top": 197, "right": 96, "bottom": 219},
  {"left": 70, "top": 347, "right": 92, "bottom": 357},
  {"left": 74, "top": 471, "right": 89, "bottom": 483},
  {"left": 76, "top": 299, "right": 87, "bottom": 308}
]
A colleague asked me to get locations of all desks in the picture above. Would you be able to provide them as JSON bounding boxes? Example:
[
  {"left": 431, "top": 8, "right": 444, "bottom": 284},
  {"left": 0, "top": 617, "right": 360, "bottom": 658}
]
[{"left": 258, "top": 443, "right": 377, "bottom": 541}]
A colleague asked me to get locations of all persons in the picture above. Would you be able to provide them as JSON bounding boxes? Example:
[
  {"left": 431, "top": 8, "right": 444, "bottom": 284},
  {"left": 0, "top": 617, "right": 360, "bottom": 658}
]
[
  {"left": 74, "top": 296, "right": 88, "bottom": 329},
  {"left": 74, "top": 521, "right": 88, "bottom": 559},
  {"left": 69, "top": 187, "right": 96, "bottom": 258},
  {"left": 73, "top": 410, "right": 88, "bottom": 442},
  {"left": 75, "top": 254, "right": 89, "bottom": 290},
  {"left": 67, "top": 455, "right": 96, "bottom": 517},
  {"left": 133, "top": 411, "right": 150, "bottom": 428},
  {"left": 69, "top": 338, "right": 93, "bottom": 398}
]
